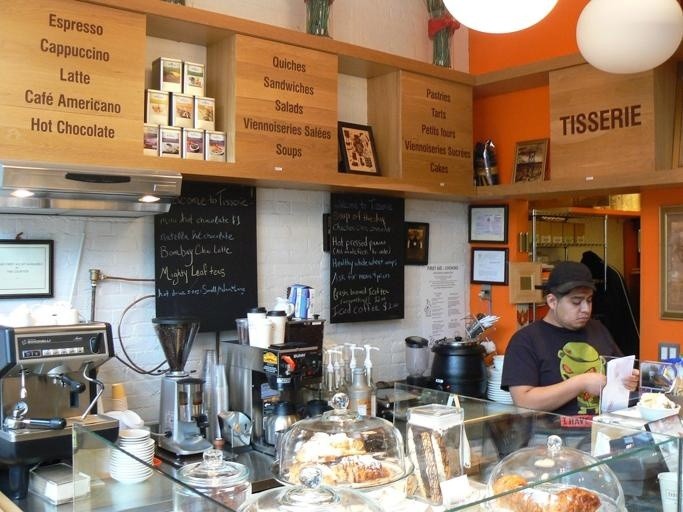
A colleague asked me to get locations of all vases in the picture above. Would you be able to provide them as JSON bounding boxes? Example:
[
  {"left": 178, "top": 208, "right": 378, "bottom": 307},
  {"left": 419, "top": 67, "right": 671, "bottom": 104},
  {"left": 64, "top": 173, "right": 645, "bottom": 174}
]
[
  {"left": 305, "top": 0, "right": 333, "bottom": 40},
  {"left": 429, "top": 10, "right": 452, "bottom": 69}
]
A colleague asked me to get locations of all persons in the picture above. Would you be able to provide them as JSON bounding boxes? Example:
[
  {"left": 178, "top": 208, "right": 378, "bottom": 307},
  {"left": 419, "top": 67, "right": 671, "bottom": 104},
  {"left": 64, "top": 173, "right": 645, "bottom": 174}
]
[{"left": 499, "top": 261, "right": 639, "bottom": 417}]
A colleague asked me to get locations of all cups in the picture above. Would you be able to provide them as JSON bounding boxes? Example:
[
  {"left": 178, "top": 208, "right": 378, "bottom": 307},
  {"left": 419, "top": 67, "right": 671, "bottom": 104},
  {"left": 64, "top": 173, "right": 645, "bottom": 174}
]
[
  {"left": 234, "top": 307, "right": 287, "bottom": 349},
  {"left": 111, "top": 383, "right": 128, "bottom": 412},
  {"left": 657, "top": 472, "right": 683, "bottom": 512},
  {"left": 201, "top": 349, "right": 229, "bottom": 445},
  {"left": 27, "top": 302, "right": 90, "bottom": 327}
]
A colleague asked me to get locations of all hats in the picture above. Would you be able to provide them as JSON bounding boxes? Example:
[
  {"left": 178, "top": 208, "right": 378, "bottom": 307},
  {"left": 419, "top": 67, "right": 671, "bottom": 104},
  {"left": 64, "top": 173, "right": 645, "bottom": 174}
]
[{"left": 546, "top": 260, "right": 598, "bottom": 294}]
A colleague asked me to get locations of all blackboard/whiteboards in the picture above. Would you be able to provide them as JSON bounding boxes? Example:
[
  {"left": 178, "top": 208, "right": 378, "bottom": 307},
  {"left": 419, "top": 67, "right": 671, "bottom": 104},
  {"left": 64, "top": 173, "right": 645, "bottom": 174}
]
[
  {"left": 153, "top": 181, "right": 259, "bottom": 333},
  {"left": 330, "top": 192, "right": 405, "bottom": 324}
]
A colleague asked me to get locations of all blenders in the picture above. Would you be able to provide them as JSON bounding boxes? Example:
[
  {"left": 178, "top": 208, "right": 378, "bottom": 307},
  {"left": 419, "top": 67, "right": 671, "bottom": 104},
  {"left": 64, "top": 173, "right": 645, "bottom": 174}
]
[
  {"left": 152, "top": 315, "right": 215, "bottom": 458},
  {"left": 405, "top": 336, "right": 429, "bottom": 388}
]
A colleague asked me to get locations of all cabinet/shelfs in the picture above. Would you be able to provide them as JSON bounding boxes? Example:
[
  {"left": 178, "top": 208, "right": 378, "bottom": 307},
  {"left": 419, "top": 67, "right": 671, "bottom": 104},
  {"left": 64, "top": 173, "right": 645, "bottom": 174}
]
[{"left": 526, "top": 211, "right": 608, "bottom": 306}]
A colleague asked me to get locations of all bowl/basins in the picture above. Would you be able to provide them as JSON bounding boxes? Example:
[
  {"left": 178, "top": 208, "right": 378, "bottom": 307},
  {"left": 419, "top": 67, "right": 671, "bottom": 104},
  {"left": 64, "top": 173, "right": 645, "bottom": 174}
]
[
  {"left": 637, "top": 400, "right": 680, "bottom": 421},
  {"left": 492, "top": 355, "right": 504, "bottom": 372}
]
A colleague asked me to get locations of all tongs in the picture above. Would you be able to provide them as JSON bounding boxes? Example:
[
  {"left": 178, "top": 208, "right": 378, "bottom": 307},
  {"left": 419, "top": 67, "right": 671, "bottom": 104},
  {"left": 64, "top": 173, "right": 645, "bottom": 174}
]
[{"left": 446, "top": 393, "right": 472, "bottom": 469}]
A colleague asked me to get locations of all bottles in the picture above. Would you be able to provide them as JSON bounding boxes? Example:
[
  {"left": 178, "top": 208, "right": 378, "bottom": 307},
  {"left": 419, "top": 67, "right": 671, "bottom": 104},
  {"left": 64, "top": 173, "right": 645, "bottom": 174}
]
[
  {"left": 172, "top": 449, "right": 252, "bottom": 512},
  {"left": 405, "top": 403, "right": 464, "bottom": 506},
  {"left": 347, "top": 367, "right": 372, "bottom": 416}
]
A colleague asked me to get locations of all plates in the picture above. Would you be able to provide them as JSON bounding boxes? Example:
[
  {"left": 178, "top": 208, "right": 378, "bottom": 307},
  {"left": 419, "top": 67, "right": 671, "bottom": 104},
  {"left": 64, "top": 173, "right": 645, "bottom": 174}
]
[
  {"left": 110, "top": 430, "right": 155, "bottom": 484},
  {"left": 487, "top": 366, "right": 514, "bottom": 405}
]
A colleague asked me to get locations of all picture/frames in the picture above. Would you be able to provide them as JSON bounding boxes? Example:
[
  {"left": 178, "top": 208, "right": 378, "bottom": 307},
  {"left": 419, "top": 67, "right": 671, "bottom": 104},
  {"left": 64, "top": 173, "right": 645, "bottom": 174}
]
[
  {"left": 511, "top": 137, "right": 548, "bottom": 183},
  {"left": 403, "top": 221, "right": 429, "bottom": 265},
  {"left": 508, "top": 262, "right": 542, "bottom": 304},
  {"left": 336, "top": 121, "right": 382, "bottom": 175},
  {"left": 658, "top": 206, "right": 683, "bottom": 320}
]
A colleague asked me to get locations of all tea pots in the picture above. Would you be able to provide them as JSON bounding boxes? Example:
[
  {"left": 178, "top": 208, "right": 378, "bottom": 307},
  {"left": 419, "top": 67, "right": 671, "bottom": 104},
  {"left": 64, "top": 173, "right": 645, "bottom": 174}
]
[
  {"left": 262, "top": 400, "right": 308, "bottom": 446},
  {"left": 220, "top": 313, "right": 325, "bottom": 457}
]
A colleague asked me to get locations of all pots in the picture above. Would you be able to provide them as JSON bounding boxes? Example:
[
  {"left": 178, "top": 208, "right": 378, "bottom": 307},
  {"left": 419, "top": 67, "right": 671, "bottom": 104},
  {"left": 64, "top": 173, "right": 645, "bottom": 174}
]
[{"left": 431, "top": 336, "right": 488, "bottom": 399}]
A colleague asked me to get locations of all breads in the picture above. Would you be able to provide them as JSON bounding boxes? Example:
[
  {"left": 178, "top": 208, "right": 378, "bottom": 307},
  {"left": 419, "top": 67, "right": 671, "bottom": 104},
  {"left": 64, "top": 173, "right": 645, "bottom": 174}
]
[
  {"left": 494, "top": 475, "right": 601, "bottom": 511},
  {"left": 286, "top": 428, "right": 390, "bottom": 486},
  {"left": 406, "top": 427, "right": 459, "bottom": 506}
]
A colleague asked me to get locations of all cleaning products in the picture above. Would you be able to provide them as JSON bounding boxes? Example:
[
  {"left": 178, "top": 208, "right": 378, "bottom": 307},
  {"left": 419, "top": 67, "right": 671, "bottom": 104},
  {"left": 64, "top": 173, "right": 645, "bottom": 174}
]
[{"left": 322, "top": 342, "right": 378, "bottom": 418}]
